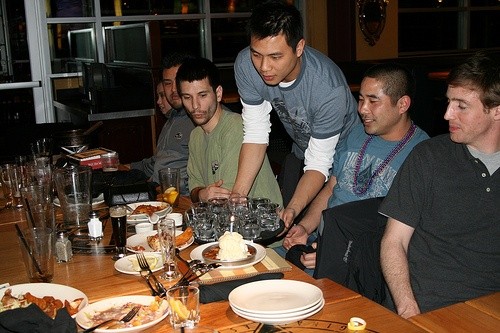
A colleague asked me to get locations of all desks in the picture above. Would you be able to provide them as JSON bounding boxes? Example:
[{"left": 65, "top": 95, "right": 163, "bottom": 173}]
[{"left": 0, "top": 156, "right": 500, "bottom": 333}]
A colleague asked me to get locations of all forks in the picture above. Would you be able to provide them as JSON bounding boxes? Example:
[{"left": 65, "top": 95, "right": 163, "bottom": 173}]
[
  {"left": 80, "top": 304, "right": 140, "bottom": 333},
  {"left": 137, "top": 250, "right": 167, "bottom": 295}
]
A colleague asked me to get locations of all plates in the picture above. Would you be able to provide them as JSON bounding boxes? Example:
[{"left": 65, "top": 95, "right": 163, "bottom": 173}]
[
  {"left": 114, "top": 251, "right": 170, "bottom": 275},
  {"left": 189, "top": 240, "right": 267, "bottom": 268},
  {"left": 124, "top": 229, "right": 194, "bottom": 254},
  {"left": 0, "top": 282, "right": 89, "bottom": 325},
  {"left": 122, "top": 201, "right": 172, "bottom": 225},
  {"left": 54, "top": 194, "right": 104, "bottom": 210},
  {"left": 72, "top": 295, "right": 172, "bottom": 332},
  {"left": 228, "top": 279, "right": 326, "bottom": 325}
]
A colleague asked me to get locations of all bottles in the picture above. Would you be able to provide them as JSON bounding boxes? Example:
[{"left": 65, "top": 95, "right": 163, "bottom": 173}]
[
  {"left": 87, "top": 211, "right": 103, "bottom": 241},
  {"left": 53, "top": 229, "right": 74, "bottom": 264}
]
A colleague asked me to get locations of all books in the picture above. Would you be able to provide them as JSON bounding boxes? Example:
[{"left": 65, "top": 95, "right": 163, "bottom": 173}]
[{"left": 66, "top": 147, "right": 116, "bottom": 169}]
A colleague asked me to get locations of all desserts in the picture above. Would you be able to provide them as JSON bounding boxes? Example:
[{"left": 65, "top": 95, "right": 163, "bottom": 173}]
[{"left": 216, "top": 231, "right": 252, "bottom": 261}]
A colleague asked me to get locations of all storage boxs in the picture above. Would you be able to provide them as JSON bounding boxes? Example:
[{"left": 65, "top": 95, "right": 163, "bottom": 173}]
[{"left": 66, "top": 147, "right": 117, "bottom": 168}]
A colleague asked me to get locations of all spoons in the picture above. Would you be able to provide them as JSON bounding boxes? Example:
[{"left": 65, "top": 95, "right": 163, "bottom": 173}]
[{"left": 170, "top": 259, "right": 220, "bottom": 290}]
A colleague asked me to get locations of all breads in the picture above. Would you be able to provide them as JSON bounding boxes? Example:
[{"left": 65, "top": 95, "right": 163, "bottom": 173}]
[
  {"left": 147, "top": 226, "right": 192, "bottom": 251},
  {"left": 1, "top": 289, "right": 84, "bottom": 319},
  {"left": 132, "top": 205, "right": 162, "bottom": 215}
]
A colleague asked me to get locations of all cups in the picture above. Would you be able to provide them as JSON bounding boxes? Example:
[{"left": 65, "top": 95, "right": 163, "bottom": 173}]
[
  {"left": 100, "top": 154, "right": 120, "bottom": 172},
  {"left": 109, "top": 206, "right": 128, "bottom": 254},
  {"left": 158, "top": 168, "right": 180, "bottom": 208},
  {"left": 157, "top": 217, "right": 176, "bottom": 259},
  {"left": 185, "top": 194, "right": 282, "bottom": 243},
  {"left": 0, "top": 137, "right": 92, "bottom": 283},
  {"left": 166, "top": 286, "right": 200, "bottom": 329}
]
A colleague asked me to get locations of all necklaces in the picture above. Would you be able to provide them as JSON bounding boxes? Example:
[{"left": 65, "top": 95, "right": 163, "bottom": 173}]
[{"left": 353, "top": 120, "right": 417, "bottom": 197}]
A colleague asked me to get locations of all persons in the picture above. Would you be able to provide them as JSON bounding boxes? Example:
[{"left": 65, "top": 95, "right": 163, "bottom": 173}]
[
  {"left": 123, "top": 55, "right": 197, "bottom": 197},
  {"left": 272, "top": 64, "right": 430, "bottom": 279},
  {"left": 176, "top": 56, "right": 289, "bottom": 248},
  {"left": 376, "top": 54, "right": 500, "bottom": 319},
  {"left": 156, "top": 81, "right": 173, "bottom": 118},
  {"left": 225, "top": 2, "right": 361, "bottom": 238}
]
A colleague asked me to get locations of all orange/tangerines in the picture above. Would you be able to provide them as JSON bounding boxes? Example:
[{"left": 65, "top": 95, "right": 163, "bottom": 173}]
[
  {"left": 171, "top": 301, "right": 189, "bottom": 319},
  {"left": 164, "top": 188, "right": 178, "bottom": 204}
]
